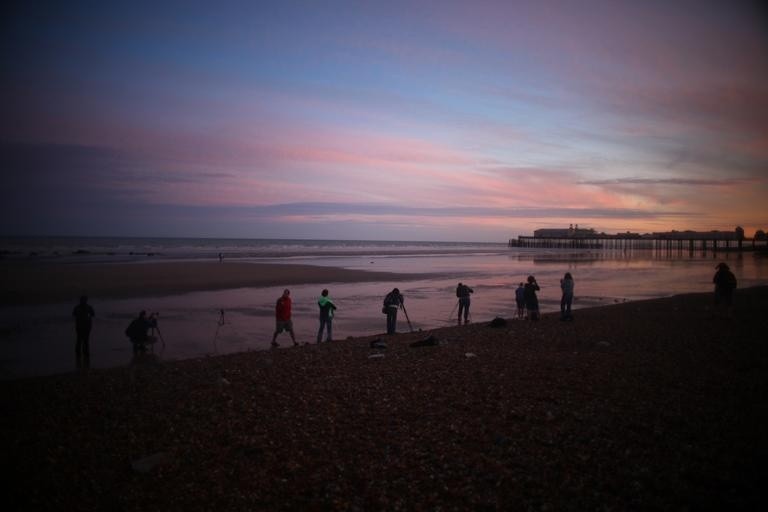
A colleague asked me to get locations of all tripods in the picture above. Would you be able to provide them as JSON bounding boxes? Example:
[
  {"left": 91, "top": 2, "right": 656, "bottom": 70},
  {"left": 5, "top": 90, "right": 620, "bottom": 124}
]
[
  {"left": 213, "top": 312, "right": 234, "bottom": 341},
  {"left": 450, "top": 301, "right": 472, "bottom": 321},
  {"left": 143, "top": 313, "right": 166, "bottom": 356}
]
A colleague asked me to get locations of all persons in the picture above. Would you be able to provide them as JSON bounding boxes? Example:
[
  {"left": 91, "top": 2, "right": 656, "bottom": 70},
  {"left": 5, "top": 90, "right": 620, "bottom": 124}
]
[
  {"left": 270, "top": 288, "right": 299, "bottom": 346},
  {"left": 515, "top": 281, "right": 525, "bottom": 320},
  {"left": 558, "top": 272, "right": 574, "bottom": 314},
  {"left": 71, "top": 292, "right": 96, "bottom": 369},
  {"left": 316, "top": 288, "right": 337, "bottom": 345},
  {"left": 522, "top": 275, "right": 541, "bottom": 319},
  {"left": 456, "top": 283, "right": 473, "bottom": 323},
  {"left": 713, "top": 263, "right": 738, "bottom": 320},
  {"left": 123, "top": 309, "right": 157, "bottom": 353},
  {"left": 383, "top": 287, "right": 404, "bottom": 334}
]
